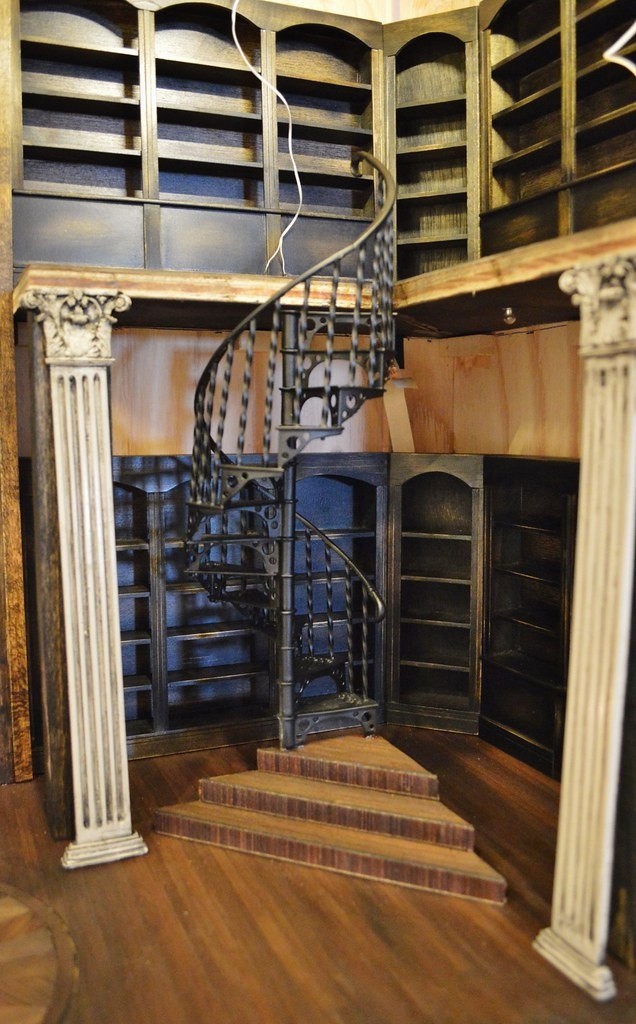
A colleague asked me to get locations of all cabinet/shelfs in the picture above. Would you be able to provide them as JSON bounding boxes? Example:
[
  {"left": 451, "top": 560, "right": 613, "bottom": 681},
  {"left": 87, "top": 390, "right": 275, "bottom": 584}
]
[
  {"left": 480, "top": 454, "right": 581, "bottom": 778},
  {"left": 479, "top": 0, "right": 636, "bottom": 259},
  {"left": 384, "top": 4, "right": 481, "bottom": 285},
  {"left": 113, "top": 453, "right": 388, "bottom": 760},
  {"left": 388, "top": 453, "right": 483, "bottom": 735},
  {"left": 11, "top": 0, "right": 383, "bottom": 279}
]
